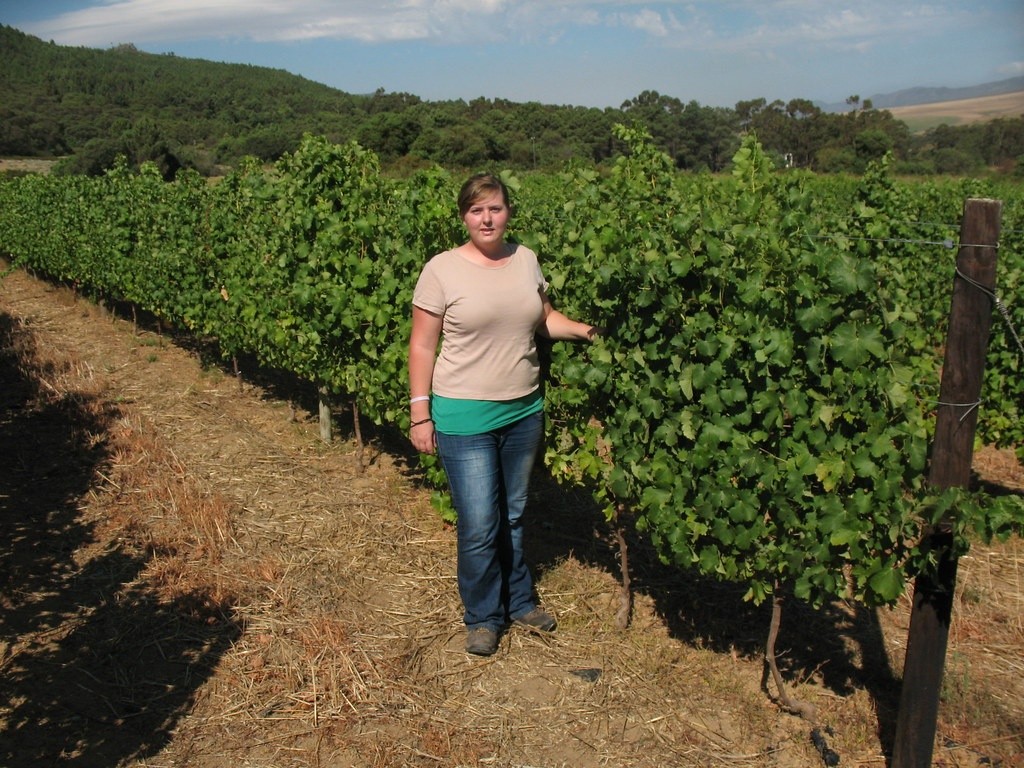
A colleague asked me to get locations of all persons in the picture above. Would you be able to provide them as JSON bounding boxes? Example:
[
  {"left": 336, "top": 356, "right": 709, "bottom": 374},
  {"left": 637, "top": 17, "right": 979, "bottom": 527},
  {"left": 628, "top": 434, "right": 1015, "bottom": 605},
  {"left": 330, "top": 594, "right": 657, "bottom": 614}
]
[{"left": 409, "top": 174, "right": 596, "bottom": 655}]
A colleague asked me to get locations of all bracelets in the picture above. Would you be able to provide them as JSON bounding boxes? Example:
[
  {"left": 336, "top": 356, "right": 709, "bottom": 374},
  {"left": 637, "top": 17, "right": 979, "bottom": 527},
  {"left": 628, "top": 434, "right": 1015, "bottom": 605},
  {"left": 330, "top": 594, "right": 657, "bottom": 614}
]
[
  {"left": 410, "top": 419, "right": 431, "bottom": 428},
  {"left": 410, "top": 396, "right": 430, "bottom": 404}
]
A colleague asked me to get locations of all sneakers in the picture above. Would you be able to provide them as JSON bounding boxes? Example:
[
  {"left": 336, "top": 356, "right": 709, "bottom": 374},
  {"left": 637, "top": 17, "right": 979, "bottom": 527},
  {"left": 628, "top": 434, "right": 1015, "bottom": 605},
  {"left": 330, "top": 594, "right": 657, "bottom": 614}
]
[
  {"left": 517, "top": 611, "right": 556, "bottom": 636},
  {"left": 468, "top": 627, "right": 499, "bottom": 656}
]
